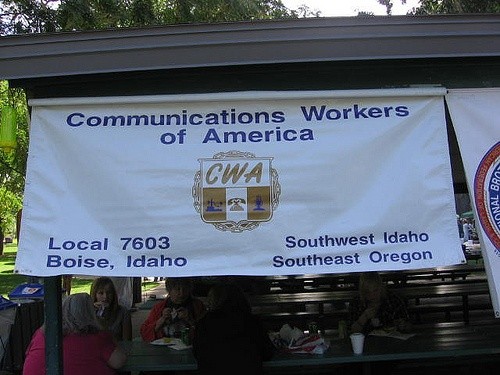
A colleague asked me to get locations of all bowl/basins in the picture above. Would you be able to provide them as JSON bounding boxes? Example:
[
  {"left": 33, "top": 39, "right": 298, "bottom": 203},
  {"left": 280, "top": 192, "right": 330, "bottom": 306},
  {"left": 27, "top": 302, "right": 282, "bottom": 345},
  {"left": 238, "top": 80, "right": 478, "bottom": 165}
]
[{"left": 474, "top": 244, "right": 481, "bottom": 249}]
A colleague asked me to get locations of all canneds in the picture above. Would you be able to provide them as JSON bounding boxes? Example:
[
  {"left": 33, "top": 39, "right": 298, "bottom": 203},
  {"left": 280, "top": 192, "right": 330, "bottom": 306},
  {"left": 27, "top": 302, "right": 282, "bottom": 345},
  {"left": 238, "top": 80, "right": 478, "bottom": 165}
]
[
  {"left": 181, "top": 327, "right": 192, "bottom": 346},
  {"left": 309, "top": 322, "right": 318, "bottom": 334},
  {"left": 339, "top": 320, "right": 347, "bottom": 338}
]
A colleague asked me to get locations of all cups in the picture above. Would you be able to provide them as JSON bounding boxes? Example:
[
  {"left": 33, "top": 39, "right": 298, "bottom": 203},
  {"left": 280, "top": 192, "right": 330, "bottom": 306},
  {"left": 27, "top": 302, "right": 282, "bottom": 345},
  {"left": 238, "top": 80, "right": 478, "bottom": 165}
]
[
  {"left": 309, "top": 321, "right": 318, "bottom": 336},
  {"left": 323, "top": 302, "right": 333, "bottom": 312},
  {"left": 305, "top": 304, "right": 319, "bottom": 311},
  {"left": 468, "top": 240, "right": 473, "bottom": 244},
  {"left": 351, "top": 333, "right": 364, "bottom": 354}
]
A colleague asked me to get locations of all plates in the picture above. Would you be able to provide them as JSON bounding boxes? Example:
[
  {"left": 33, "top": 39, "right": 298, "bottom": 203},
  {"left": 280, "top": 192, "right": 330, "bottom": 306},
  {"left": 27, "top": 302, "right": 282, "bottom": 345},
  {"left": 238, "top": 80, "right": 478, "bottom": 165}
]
[{"left": 150, "top": 339, "right": 179, "bottom": 345}]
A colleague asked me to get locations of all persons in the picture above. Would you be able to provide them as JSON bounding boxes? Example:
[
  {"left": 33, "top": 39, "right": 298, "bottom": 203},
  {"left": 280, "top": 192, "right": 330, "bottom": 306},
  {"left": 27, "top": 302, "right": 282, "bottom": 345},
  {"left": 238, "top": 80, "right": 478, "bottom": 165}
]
[
  {"left": 24, "top": 292, "right": 128, "bottom": 375},
  {"left": 89, "top": 268, "right": 387, "bottom": 375},
  {"left": 456, "top": 215, "right": 478, "bottom": 243}
]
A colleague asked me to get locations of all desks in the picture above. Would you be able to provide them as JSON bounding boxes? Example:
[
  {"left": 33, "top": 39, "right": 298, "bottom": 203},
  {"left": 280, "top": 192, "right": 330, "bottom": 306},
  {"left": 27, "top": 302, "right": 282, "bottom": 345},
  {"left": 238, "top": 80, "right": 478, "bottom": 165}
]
[
  {"left": 112, "top": 321, "right": 499, "bottom": 375},
  {"left": 139, "top": 265, "right": 490, "bottom": 320}
]
